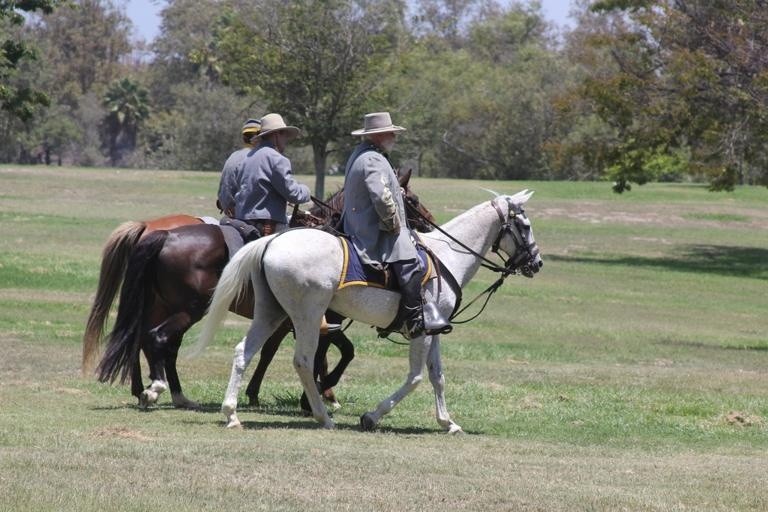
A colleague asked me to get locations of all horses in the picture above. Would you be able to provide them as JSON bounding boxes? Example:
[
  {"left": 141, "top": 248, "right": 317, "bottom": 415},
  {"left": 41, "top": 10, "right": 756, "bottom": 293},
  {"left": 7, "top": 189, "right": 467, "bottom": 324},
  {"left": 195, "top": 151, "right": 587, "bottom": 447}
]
[
  {"left": 191, "top": 188, "right": 545, "bottom": 435},
  {"left": 84, "top": 186, "right": 345, "bottom": 404},
  {"left": 94, "top": 167, "right": 437, "bottom": 413}
]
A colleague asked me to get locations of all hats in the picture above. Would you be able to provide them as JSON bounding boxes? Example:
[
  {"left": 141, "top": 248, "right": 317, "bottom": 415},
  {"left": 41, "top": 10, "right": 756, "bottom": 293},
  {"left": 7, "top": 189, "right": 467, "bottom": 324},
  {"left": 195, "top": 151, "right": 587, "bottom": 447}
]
[
  {"left": 242, "top": 112, "right": 299, "bottom": 141},
  {"left": 351, "top": 112, "right": 407, "bottom": 136}
]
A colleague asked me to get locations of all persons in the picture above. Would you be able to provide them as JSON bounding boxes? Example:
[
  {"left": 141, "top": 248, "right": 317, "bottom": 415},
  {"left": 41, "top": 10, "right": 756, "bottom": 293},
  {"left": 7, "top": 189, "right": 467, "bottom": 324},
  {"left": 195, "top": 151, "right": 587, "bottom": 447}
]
[
  {"left": 340, "top": 111, "right": 450, "bottom": 338},
  {"left": 217, "top": 113, "right": 342, "bottom": 331}
]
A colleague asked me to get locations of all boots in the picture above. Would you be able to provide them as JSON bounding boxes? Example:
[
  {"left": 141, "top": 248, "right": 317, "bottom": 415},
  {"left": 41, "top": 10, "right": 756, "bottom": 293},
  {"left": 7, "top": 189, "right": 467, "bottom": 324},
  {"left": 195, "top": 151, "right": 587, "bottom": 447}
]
[{"left": 402, "top": 270, "right": 453, "bottom": 338}]
